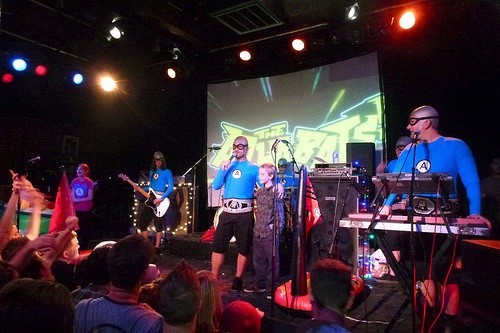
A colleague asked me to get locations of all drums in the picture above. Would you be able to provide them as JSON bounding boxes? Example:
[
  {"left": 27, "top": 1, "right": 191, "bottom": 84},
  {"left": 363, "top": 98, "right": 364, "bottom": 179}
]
[
  {"left": 213, "top": 206, "right": 236, "bottom": 244},
  {"left": 284, "top": 187, "right": 298, "bottom": 201}
]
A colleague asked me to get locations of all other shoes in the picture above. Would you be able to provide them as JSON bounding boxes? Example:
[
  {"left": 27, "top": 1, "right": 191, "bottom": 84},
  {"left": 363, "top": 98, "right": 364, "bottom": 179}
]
[
  {"left": 243, "top": 284, "right": 267, "bottom": 293},
  {"left": 230, "top": 277, "right": 244, "bottom": 293},
  {"left": 154, "top": 246, "right": 164, "bottom": 255},
  {"left": 264, "top": 290, "right": 275, "bottom": 300}
]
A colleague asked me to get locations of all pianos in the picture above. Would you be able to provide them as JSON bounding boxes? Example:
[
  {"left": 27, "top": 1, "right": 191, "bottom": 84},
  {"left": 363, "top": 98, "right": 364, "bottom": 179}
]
[{"left": 338, "top": 173, "right": 490, "bottom": 333}]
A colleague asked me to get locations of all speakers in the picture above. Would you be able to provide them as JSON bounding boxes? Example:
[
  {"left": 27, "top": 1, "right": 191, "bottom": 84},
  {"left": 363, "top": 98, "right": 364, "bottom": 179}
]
[
  {"left": 310, "top": 179, "right": 358, "bottom": 270},
  {"left": 345, "top": 142, "right": 378, "bottom": 196}
]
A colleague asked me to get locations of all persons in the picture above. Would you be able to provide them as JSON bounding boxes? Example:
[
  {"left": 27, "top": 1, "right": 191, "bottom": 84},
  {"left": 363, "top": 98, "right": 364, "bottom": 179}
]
[
  {"left": 376, "top": 106, "right": 491, "bottom": 333},
  {"left": 0, "top": 135, "right": 411, "bottom": 333}
]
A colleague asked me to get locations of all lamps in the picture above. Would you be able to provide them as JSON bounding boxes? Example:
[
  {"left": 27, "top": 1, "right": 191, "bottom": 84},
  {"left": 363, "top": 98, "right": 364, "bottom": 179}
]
[{"left": 165, "top": 41, "right": 194, "bottom": 80}]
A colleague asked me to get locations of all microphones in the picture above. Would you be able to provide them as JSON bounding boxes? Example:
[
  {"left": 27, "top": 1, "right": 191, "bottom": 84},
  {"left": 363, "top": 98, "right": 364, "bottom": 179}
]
[
  {"left": 27, "top": 156, "right": 41, "bottom": 163},
  {"left": 230, "top": 153, "right": 237, "bottom": 161},
  {"left": 207, "top": 147, "right": 222, "bottom": 150},
  {"left": 414, "top": 130, "right": 421, "bottom": 136},
  {"left": 272, "top": 136, "right": 282, "bottom": 149}
]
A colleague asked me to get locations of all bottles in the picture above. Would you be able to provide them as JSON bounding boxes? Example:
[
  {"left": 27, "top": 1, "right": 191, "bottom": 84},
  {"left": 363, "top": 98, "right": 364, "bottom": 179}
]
[{"left": 333, "top": 150, "right": 339, "bottom": 163}]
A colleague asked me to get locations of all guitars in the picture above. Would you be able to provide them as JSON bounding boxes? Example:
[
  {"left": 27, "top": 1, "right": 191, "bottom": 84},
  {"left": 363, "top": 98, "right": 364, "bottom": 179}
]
[{"left": 118, "top": 173, "right": 171, "bottom": 218}]
